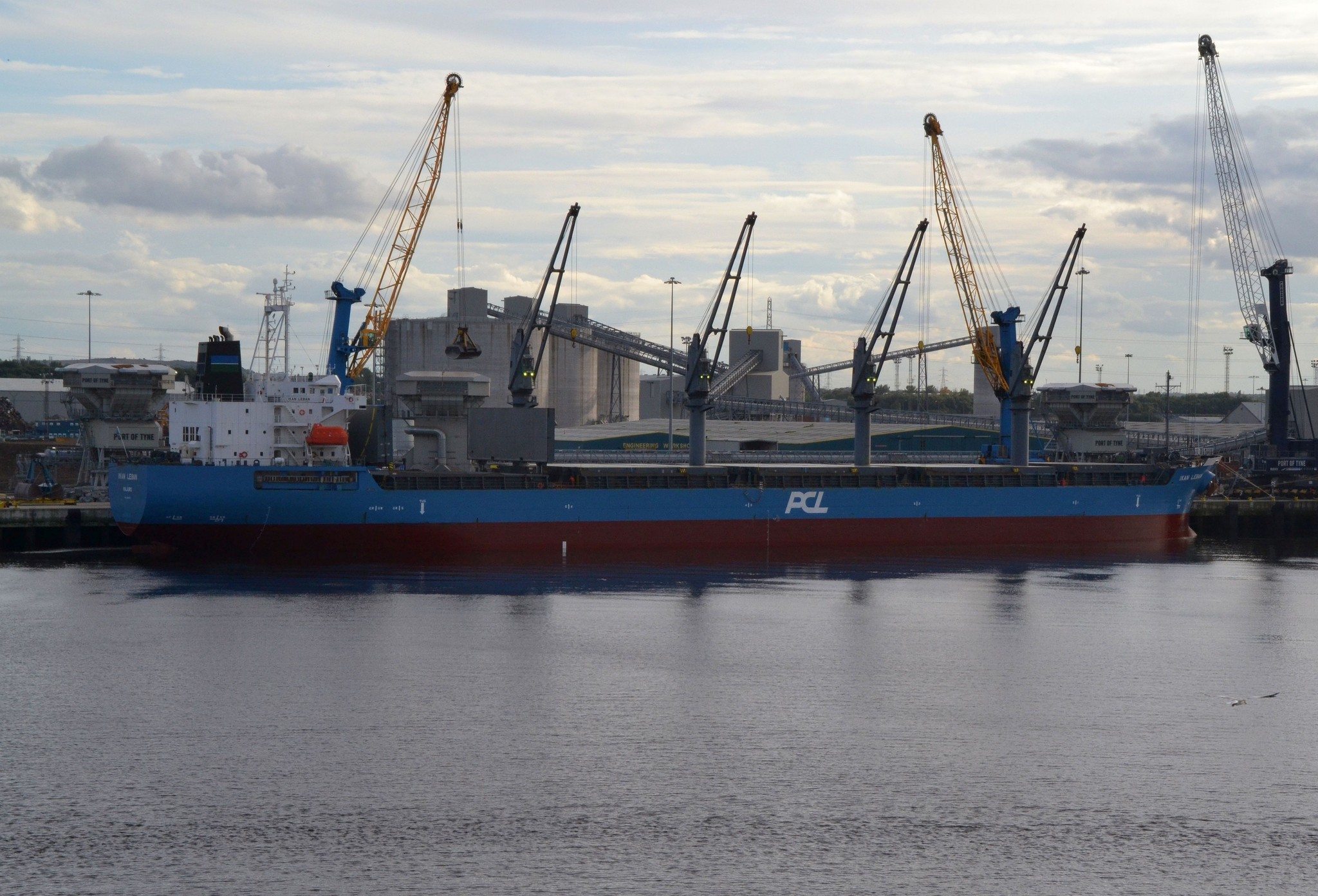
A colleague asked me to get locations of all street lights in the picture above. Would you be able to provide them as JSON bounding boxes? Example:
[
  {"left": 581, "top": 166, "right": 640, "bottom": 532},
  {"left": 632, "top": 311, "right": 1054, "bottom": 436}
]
[
  {"left": 76, "top": 290, "right": 103, "bottom": 363},
  {"left": 39, "top": 372, "right": 55, "bottom": 438},
  {"left": 1310, "top": 360, "right": 1318, "bottom": 386},
  {"left": 1223, "top": 345, "right": 1234, "bottom": 424},
  {"left": 1125, "top": 354, "right": 1133, "bottom": 384},
  {"left": 1255, "top": 386, "right": 1267, "bottom": 422},
  {"left": 664, "top": 277, "right": 682, "bottom": 454},
  {"left": 892, "top": 357, "right": 902, "bottom": 410},
  {"left": 1096, "top": 364, "right": 1104, "bottom": 383}
]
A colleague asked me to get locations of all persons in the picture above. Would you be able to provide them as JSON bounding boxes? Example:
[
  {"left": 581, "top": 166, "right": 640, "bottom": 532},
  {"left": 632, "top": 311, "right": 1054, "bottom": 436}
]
[{"left": 978, "top": 452, "right": 986, "bottom": 464}]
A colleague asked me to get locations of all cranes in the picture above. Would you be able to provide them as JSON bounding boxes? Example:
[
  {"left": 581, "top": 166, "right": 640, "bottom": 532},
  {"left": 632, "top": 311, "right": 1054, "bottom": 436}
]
[
  {"left": 1197, "top": 34, "right": 1291, "bottom": 485},
  {"left": 326, "top": 74, "right": 467, "bottom": 392},
  {"left": 921, "top": 113, "right": 1023, "bottom": 464}
]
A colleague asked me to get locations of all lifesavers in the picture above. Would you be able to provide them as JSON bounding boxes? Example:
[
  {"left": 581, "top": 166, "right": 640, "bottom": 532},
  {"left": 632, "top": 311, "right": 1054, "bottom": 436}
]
[
  {"left": 299, "top": 409, "right": 305, "bottom": 416},
  {"left": 241, "top": 451, "right": 248, "bottom": 458},
  {"left": 537, "top": 483, "right": 543, "bottom": 489},
  {"left": 348, "top": 397, "right": 354, "bottom": 402}
]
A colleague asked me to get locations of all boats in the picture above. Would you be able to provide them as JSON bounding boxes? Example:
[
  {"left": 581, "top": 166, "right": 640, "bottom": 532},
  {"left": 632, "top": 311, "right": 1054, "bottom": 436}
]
[
  {"left": 306, "top": 423, "right": 348, "bottom": 445},
  {"left": 103, "top": 201, "right": 1222, "bottom": 570}
]
